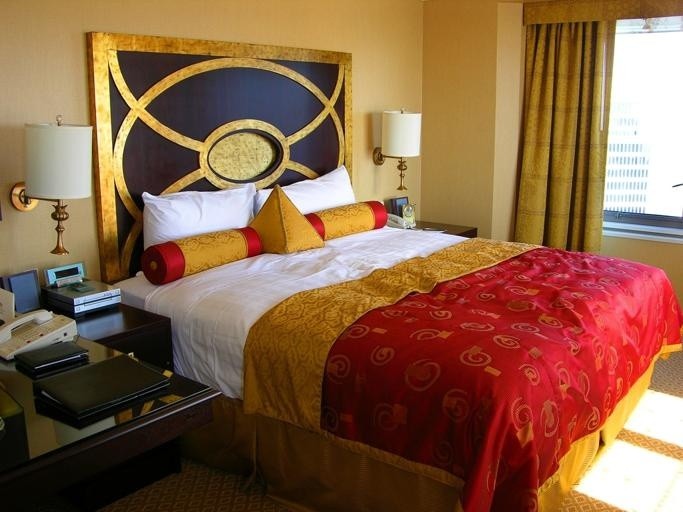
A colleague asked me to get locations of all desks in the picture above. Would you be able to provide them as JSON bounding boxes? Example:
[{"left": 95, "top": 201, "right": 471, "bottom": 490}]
[{"left": 1, "top": 308, "right": 224, "bottom": 512}]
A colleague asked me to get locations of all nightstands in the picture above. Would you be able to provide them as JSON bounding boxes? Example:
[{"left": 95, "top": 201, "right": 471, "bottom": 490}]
[
  {"left": 26, "top": 295, "right": 175, "bottom": 376},
  {"left": 410, "top": 219, "right": 478, "bottom": 238}
]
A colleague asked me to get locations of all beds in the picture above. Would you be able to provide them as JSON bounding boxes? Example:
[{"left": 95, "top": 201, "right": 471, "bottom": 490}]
[{"left": 83, "top": 27, "right": 683, "bottom": 512}]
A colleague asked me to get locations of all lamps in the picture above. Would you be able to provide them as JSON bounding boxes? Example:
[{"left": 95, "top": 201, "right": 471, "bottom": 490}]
[
  {"left": 9, "top": 113, "right": 95, "bottom": 257},
  {"left": 372, "top": 107, "right": 423, "bottom": 191}
]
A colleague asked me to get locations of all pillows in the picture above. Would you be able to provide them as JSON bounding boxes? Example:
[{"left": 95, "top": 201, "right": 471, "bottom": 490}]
[
  {"left": 247, "top": 183, "right": 326, "bottom": 255},
  {"left": 302, "top": 200, "right": 389, "bottom": 241},
  {"left": 253, "top": 165, "right": 356, "bottom": 216},
  {"left": 139, "top": 226, "right": 264, "bottom": 287},
  {"left": 139, "top": 181, "right": 256, "bottom": 249}
]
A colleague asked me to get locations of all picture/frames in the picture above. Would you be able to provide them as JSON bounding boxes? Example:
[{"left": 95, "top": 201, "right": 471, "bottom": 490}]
[{"left": 390, "top": 195, "right": 410, "bottom": 226}]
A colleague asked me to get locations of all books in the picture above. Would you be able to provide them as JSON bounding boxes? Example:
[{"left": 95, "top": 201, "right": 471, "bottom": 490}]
[
  {"left": 14, "top": 341, "right": 89, "bottom": 374},
  {"left": 13, "top": 362, "right": 92, "bottom": 380}
]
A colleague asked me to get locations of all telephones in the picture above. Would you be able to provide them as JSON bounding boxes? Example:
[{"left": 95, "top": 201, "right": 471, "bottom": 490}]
[
  {"left": 1, "top": 309, "right": 78, "bottom": 361},
  {"left": 386, "top": 213, "right": 407, "bottom": 230}
]
